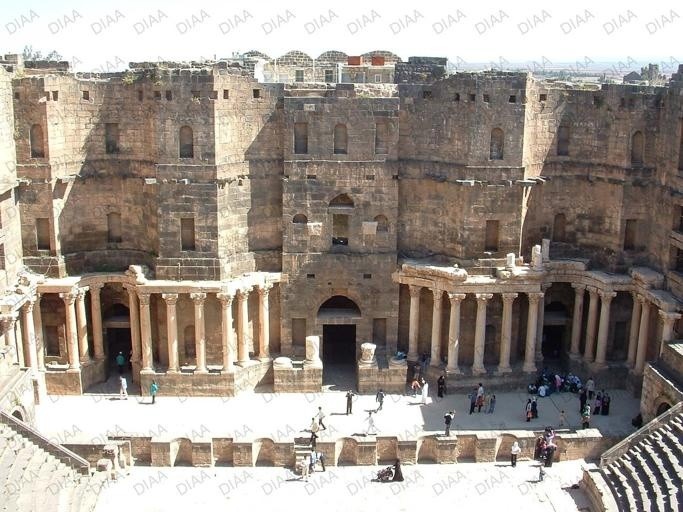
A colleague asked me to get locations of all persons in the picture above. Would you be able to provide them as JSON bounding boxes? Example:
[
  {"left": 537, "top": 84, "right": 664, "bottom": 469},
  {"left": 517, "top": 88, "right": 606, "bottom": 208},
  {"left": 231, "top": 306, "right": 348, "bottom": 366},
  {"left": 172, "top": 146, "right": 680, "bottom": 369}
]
[
  {"left": 300, "top": 369, "right": 611, "bottom": 482},
  {"left": 150, "top": 382, "right": 158, "bottom": 403},
  {"left": 118, "top": 375, "right": 128, "bottom": 400},
  {"left": 116, "top": 352, "right": 124, "bottom": 373}
]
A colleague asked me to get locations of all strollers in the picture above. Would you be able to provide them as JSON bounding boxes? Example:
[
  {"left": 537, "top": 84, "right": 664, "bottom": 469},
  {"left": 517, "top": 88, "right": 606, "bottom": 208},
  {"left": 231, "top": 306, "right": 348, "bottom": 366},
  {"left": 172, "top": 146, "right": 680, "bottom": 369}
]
[{"left": 376, "top": 466, "right": 394, "bottom": 482}]
